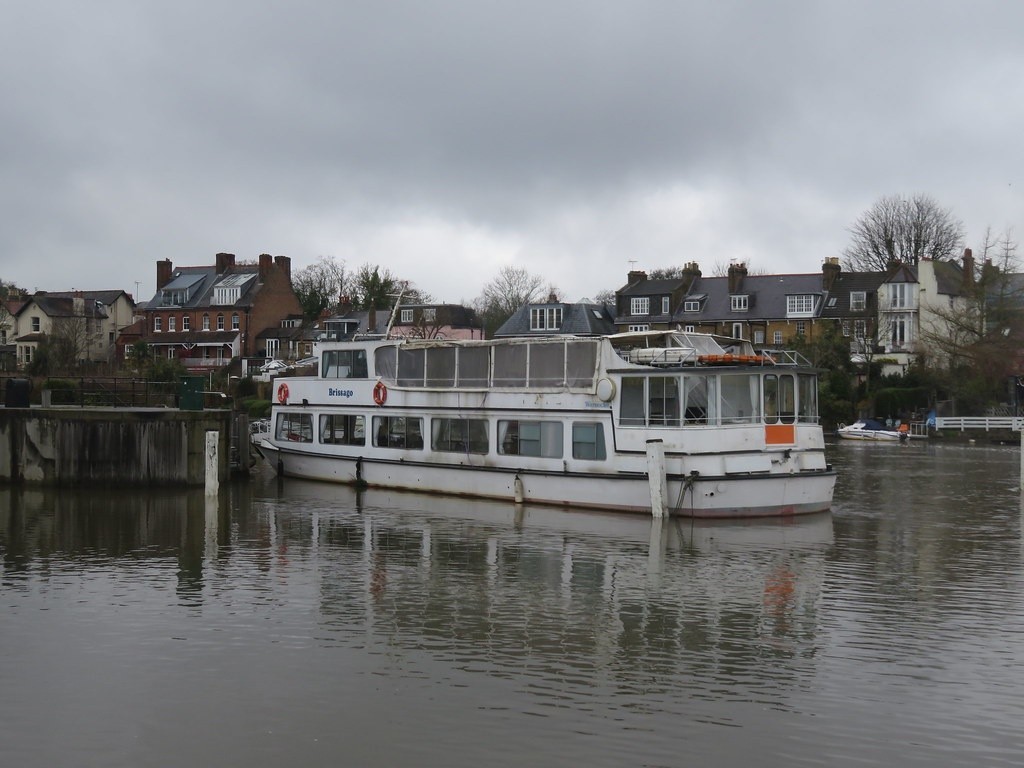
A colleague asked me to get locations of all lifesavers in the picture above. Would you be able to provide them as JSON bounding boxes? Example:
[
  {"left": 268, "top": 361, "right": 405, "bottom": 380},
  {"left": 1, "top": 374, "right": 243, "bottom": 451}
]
[
  {"left": 278, "top": 383, "right": 288, "bottom": 403},
  {"left": 373, "top": 383, "right": 387, "bottom": 405}
]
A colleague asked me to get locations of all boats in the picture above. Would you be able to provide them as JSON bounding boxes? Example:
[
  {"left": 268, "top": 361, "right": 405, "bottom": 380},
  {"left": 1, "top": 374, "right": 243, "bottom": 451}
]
[
  {"left": 837, "top": 420, "right": 911, "bottom": 443},
  {"left": 248, "top": 282, "right": 840, "bottom": 521}
]
